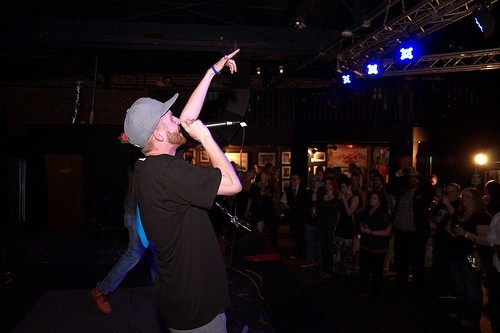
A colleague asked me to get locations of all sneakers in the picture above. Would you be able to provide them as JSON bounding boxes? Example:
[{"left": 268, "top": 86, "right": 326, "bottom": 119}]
[{"left": 91, "top": 287, "right": 112, "bottom": 314}]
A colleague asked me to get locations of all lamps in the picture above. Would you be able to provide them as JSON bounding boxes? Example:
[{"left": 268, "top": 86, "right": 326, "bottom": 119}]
[{"left": 474, "top": 0, "right": 495, "bottom": 38}]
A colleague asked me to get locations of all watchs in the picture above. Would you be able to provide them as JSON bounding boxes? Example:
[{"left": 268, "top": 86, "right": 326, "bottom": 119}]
[{"left": 464, "top": 231, "right": 469, "bottom": 238}]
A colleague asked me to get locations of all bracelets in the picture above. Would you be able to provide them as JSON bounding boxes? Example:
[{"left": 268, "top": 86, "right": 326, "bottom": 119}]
[
  {"left": 370, "top": 230, "right": 373, "bottom": 235},
  {"left": 211, "top": 65, "right": 220, "bottom": 76},
  {"left": 474, "top": 235, "right": 478, "bottom": 243}
]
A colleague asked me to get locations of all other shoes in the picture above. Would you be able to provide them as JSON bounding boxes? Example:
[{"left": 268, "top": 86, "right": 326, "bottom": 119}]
[
  {"left": 461, "top": 320, "right": 475, "bottom": 325},
  {"left": 449, "top": 313, "right": 459, "bottom": 317},
  {"left": 290, "top": 254, "right": 300, "bottom": 259},
  {"left": 300, "top": 262, "right": 314, "bottom": 267}
]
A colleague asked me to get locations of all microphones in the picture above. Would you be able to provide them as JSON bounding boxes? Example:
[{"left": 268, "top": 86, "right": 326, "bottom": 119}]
[{"left": 179, "top": 121, "right": 233, "bottom": 137}]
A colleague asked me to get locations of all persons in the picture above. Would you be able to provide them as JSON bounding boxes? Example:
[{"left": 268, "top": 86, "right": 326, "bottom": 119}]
[
  {"left": 92, "top": 153, "right": 149, "bottom": 314},
  {"left": 186, "top": 154, "right": 500, "bottom": 320},
  {"left": 124, "top": 49, "right": 240, "bottom": 333}
]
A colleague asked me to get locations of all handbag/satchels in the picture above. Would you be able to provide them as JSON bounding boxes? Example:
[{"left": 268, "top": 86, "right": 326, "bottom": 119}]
[
  {"left": 478, "top": 260, "right": 494, "bottom": 287},
  {"left": 468, "top": 252, "right": 481, "bottom": 269}
]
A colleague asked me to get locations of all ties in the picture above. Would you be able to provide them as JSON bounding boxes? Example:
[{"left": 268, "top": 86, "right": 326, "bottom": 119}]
[{"left": 293, "top": 186, "right": 296, "bottom": 199}]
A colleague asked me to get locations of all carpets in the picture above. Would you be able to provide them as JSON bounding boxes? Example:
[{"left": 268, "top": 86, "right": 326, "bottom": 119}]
[{"left": 11, "top": 286, "right": 163, "bottom": 333}]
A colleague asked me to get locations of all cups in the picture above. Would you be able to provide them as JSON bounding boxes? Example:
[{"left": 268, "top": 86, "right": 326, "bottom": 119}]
[{"left": 454, "top": 224, "right": 460, "bottom": 236}]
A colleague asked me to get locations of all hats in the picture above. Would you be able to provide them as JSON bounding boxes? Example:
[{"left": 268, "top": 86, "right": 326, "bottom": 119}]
[{"left": 124, "top": 93, "right": 180, "bottom": 148}]
